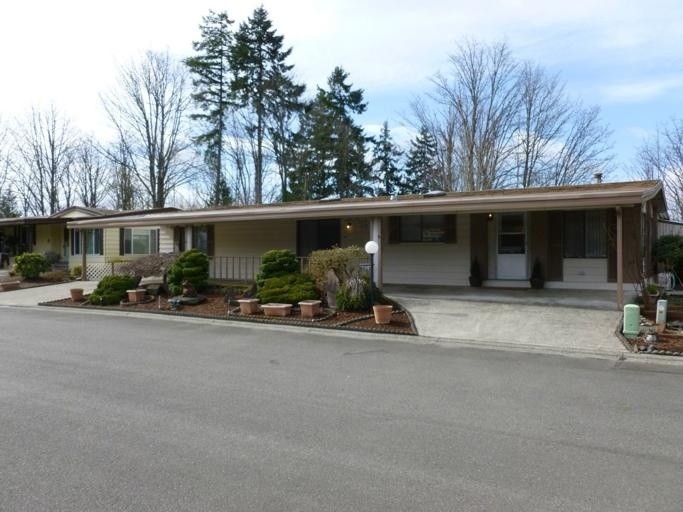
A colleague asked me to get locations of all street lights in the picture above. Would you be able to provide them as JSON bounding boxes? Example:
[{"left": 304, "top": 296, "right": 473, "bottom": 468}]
[{"left": 364, "top": 240, "right": 380, "bottom": 308}]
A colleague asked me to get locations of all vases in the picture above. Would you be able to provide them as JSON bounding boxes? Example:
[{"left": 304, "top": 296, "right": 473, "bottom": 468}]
[
  {"left": 126, "top": 288, "right": 146, "bottom": 303},
  {"left": 235, "top": 297, "right": 260, "bottom": 315},
  {"left": 372, "top": 304, "right": 393, "bottom": 325},
  {"left": 69, "top": 288, "right": 84, "bottom": 303},
  {"left": 260, "top": 302, "right": 293, "bottom": 317},
  {"left": 297, "top": 299, "right": 322, "bottom": 318}
]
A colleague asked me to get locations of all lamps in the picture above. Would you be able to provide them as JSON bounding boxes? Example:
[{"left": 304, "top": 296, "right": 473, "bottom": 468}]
[
  {"left": 346, "top": 223, "right": 351, "bottom": 229},
  {"left": 489, "top": 210, "right": 492, "bottom": 218}
]
